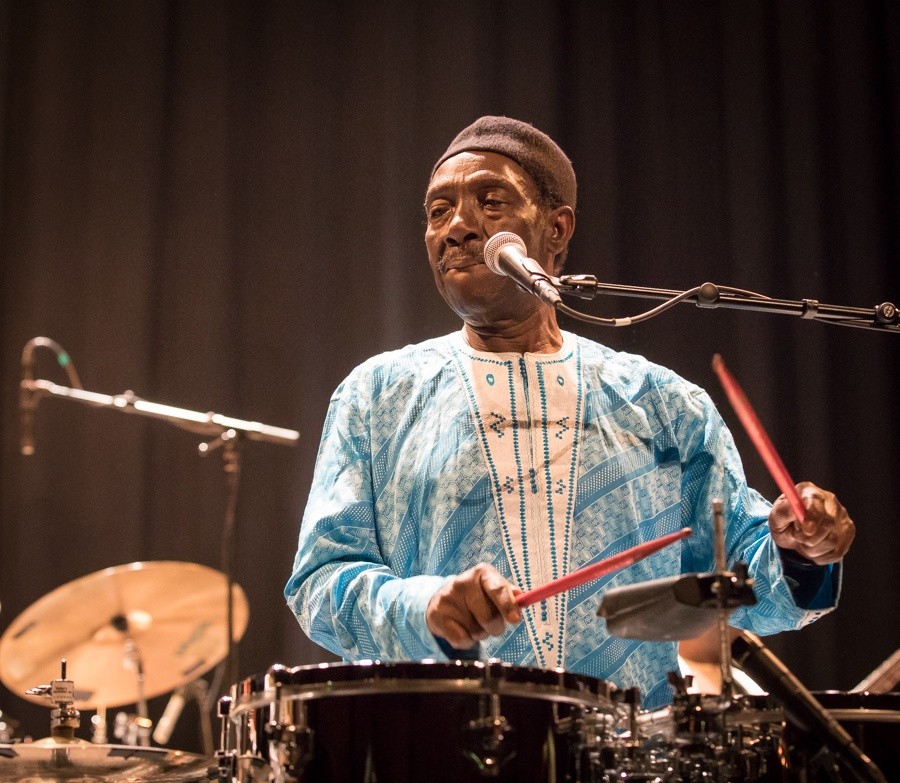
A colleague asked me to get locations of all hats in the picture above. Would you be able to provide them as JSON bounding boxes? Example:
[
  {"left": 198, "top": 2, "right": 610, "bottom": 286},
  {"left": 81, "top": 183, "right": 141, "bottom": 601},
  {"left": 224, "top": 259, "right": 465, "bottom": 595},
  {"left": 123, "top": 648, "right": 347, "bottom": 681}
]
[{"left": 430, "top": 114, "right": 578, "bottom": 215}]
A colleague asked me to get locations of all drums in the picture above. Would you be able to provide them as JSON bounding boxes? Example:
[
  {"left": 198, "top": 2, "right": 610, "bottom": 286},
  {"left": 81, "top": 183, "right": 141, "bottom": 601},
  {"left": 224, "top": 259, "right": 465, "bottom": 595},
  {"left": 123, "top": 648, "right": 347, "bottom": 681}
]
[
  {"left": 220, "top": 660, "right": 617, "bottom": 783},
  {"left": 692, "top": 695, "right": 900, "bottom": 783}
]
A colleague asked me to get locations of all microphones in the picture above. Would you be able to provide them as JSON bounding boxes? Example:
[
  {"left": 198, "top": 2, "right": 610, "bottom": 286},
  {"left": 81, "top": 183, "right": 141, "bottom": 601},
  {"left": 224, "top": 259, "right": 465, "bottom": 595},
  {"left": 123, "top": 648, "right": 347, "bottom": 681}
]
[
  {"left": 152, "top": 679, "right": 193, "bottom": 745},
  {"left": 20, "top": 349, "right": 37, "bottom": 455},
  {"left": 483, "top": 231, "right": 562, "bottom": 309},
  {"left": 730, "top": 629, "right": 888, "bottom": 783}
]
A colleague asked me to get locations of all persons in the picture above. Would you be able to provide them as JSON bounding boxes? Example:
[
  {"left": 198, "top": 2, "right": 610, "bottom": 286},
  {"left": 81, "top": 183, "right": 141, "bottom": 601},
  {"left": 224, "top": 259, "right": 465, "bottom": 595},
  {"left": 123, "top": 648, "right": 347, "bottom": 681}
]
[{"left": 283, "top": 116, "right": 856, "bottom": 714}]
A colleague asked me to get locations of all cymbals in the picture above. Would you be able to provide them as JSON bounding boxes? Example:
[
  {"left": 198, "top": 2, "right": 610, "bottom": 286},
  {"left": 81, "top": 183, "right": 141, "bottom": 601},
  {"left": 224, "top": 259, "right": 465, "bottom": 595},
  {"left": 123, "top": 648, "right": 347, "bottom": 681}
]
[
  {"left": 0, "top": 735, "right": 218, "bottom": 783},
  {"left": 0, "top": 560, "right": 251, "bottom": 711}
]
